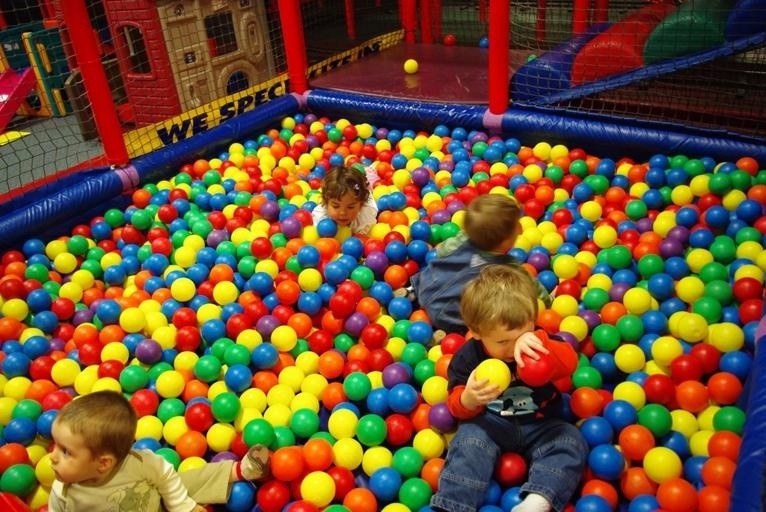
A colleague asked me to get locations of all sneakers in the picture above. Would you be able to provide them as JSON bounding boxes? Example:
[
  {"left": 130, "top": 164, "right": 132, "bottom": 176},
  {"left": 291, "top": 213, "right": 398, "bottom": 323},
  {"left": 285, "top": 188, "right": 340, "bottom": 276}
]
[{"left": 239, "top": 442, "right": 275, "bottom": 483}]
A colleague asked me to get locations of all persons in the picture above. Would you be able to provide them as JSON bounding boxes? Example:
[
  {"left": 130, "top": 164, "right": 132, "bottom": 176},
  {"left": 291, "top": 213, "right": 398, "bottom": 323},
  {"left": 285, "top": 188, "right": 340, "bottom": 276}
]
[
  {"left": 410, "top": 193, "right": 558, "bottom": 339},
  {"left": 431, "top": 264, "right": 588, "bottom": 512},
  {"left": 312, "top": 165, "right": 379, "bottom": 237},
  {"left": 48, "top": 390, "right": 272, "bottom": 511}
]
